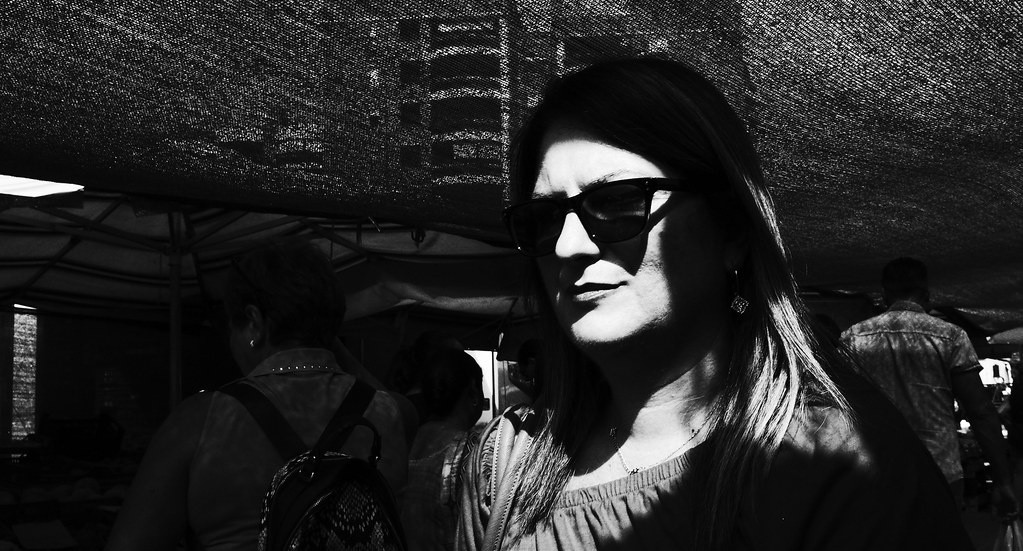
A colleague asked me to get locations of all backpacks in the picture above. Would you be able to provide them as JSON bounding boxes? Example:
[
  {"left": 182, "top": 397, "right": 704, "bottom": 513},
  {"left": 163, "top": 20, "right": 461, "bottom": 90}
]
[{"left": 215, "top": 376, "right": 407, "bottom": 551}]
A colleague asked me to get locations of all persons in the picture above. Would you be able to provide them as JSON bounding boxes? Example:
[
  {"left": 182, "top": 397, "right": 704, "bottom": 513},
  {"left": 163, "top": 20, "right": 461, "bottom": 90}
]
[
  {"left": 350, "top": 328, "right": 466, "bottom": 551},
  {"left": 453, "top": 52, "right": 974, "bottom": 551},
  {"left": 816, "top": 258, "right": 1023, "bottom": 551},
  {"left": 74, "top": 364, "right": 144, "bottom": 483},
  {"left": 106, "top": 237, "right": 417, "bottom": 551}
]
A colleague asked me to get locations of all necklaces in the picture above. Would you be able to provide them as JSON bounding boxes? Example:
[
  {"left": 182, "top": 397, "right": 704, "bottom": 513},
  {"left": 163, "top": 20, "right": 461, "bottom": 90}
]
[{"left": 607, "top": 402, "right": 709, "bottom": 476}]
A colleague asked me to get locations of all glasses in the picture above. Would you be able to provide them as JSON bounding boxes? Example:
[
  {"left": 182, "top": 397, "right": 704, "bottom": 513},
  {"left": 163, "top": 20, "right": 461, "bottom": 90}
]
[{"left": 504, "top": 176, "right": 684, "bottom": 256}]
